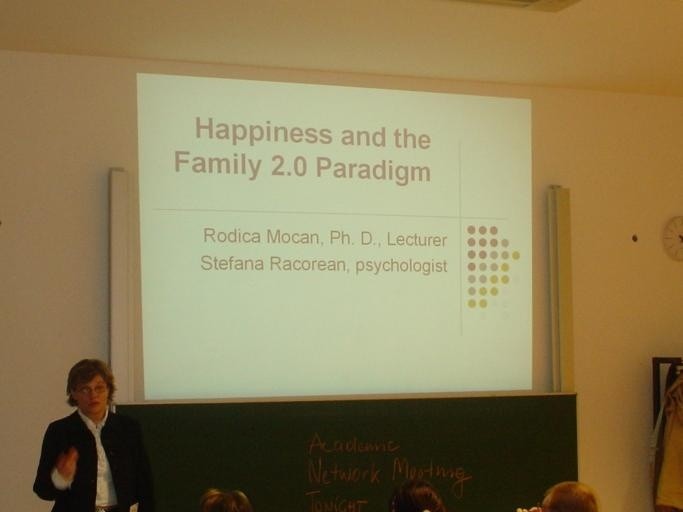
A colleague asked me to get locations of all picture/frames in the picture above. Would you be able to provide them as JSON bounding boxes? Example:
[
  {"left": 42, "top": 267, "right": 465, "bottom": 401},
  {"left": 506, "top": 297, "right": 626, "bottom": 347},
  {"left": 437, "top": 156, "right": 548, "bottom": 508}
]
[{"left": 649, "top": 356, "right": 682, "bottom": 428}]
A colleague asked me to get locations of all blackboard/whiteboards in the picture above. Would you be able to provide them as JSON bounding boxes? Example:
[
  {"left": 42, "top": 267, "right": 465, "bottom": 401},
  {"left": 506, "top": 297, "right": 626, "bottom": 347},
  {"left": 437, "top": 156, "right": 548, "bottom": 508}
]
[{"left": 114, "top": 394, "right": 579, "bottom": 511}]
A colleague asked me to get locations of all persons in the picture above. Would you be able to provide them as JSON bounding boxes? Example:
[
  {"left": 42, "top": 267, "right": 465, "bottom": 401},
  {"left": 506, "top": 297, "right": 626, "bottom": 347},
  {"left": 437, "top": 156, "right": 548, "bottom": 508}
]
[
  {"left": 388, "top": 479, "right": 447, "bottom": 512},
  {"left": 33, "top": 358, "right": 157, "bottom": 512},
  {"left": 197, "top": 487, "right": 254, "bottom": 512},
  {"left": 515, "top": 479, "right": 598, "bottom": 512}
]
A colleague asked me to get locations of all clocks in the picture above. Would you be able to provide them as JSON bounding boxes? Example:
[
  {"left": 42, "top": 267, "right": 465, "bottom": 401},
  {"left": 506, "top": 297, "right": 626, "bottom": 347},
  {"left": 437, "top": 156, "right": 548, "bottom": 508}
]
[{"left": 661, "top": 217, "right": 683, "bottom": 259}]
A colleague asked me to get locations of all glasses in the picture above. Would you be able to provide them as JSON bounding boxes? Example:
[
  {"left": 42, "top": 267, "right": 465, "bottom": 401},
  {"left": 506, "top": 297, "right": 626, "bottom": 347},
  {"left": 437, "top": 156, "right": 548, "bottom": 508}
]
[{"left": 75, "top": 384, "right": 108, "bottom": 394}]
